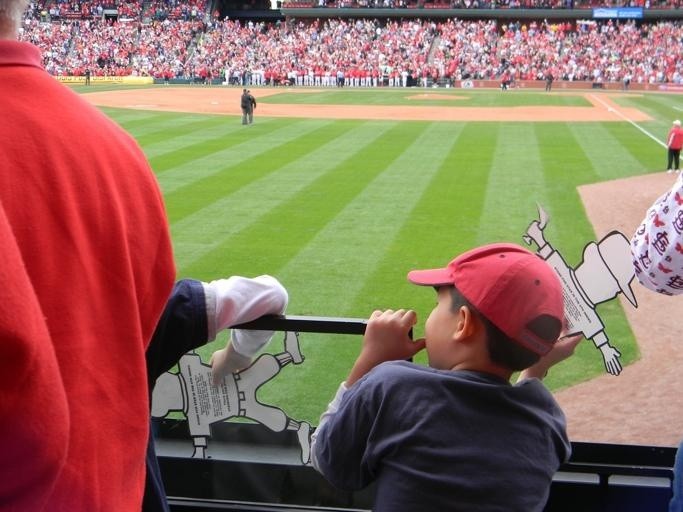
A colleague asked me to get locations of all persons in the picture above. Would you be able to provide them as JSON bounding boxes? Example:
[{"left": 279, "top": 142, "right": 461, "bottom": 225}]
[
  {"left": 307, "top": 245, "right": 587, "bottom": 512},
  {"left": 246, "top": 90, "right": 256, "bottom": 125},
  {"left": 665, "top": 119, "right": 682, "bottom": 175},
  {"left": 239, "top": 88, "right": 251, "bottom": 126},
  {"left": 0, "top": 0, "right": 682, "bottom": 92},
  {"left": 0, "top": 0, "right": 176, "bottom": 511},
  {"left": 626, "top": 160, "right": 683, "bottom": 511},
  {"left": 144, "top": 273, "right": 288, "bottom": 511}
]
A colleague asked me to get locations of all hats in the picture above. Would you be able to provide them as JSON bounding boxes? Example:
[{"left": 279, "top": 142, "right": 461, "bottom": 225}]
[{"left": 408, "top": 241, "right": 566, "bottom": 358}]
